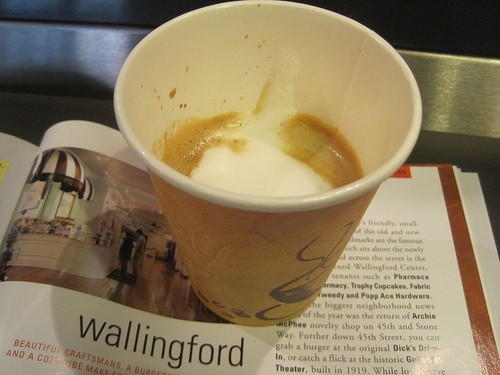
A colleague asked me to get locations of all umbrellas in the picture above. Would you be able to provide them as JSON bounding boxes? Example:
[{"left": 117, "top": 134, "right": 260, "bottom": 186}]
[{"left": 37, "top": 150, "right": 95, "bottom": 217}]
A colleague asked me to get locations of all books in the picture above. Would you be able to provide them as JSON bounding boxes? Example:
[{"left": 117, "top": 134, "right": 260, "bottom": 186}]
[{"left": 0, "top": 120, "right": 500, "bottom": 375}]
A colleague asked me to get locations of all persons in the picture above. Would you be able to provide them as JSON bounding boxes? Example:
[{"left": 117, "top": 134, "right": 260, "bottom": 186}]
[
  {"left": 117, "top": 231, "right": 134, "bottom": 283},
  {"left": 130, "top": 230, "right": 145, "bottom": 280}
]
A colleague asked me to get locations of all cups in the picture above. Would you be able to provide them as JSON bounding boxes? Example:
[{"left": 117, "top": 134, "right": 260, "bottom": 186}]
[{"left": 114, "top": 1, "right": 422, "bottom": 327}]
